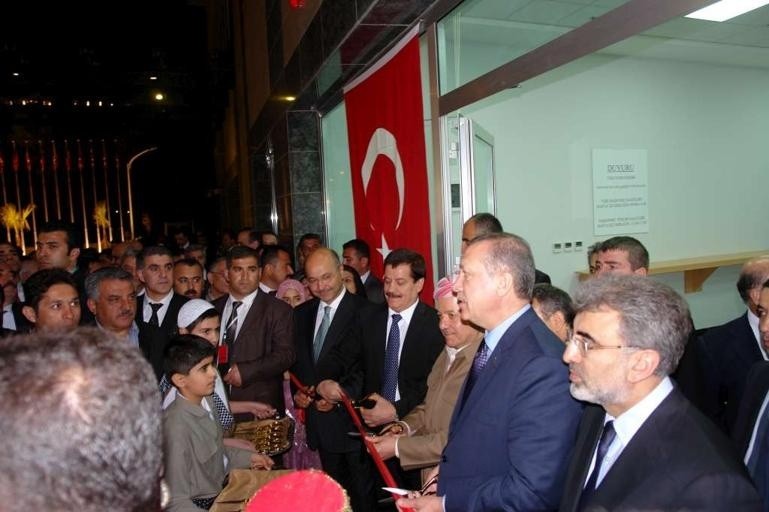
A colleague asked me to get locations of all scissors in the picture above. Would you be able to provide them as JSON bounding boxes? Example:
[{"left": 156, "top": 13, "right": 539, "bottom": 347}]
[
  {"left": 333, "top": 389, "right": 381, "bottom": 408},
  {"left": 378, "top": 474, "right": 439, "bottom": 503},
  {"left": 346, "top": 420, "right": 404, "bottom": 438}
]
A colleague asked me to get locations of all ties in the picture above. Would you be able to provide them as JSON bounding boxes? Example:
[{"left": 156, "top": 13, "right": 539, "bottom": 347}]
[
  {"left": 381, "top": 314, "right": 402, "bottom": 402},
  {"left": 313, "top": 306, "right": 332, "bottom": 368},
  {"left": 148, "top": 303, "right": 164, "bottom": 327},
  {"left": 460, "top": 338, "right": 488, "bottom": 412},
  {"left": 217, "top": 302, "right": 243, "bottom": 400},
  {"left": 577, "top": 420, "right": 616, "bottom": 512}
]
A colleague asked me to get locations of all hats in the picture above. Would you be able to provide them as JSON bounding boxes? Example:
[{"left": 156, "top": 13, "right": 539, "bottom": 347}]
[{"left": 176, "top": 297, "right": 216, "bottom": 329}]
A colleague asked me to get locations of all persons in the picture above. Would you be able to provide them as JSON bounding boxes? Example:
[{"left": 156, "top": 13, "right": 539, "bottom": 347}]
[{"left": 1, "top": 214, "right": 769, "bottom": 512}]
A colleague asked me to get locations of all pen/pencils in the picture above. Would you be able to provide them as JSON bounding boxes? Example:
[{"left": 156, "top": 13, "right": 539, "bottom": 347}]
[{"left": 216, "top": 498, "right": 249, "bottom": 503}]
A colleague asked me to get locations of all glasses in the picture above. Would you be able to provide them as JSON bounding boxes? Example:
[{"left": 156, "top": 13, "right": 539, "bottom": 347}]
[{"left": 564, "top": 329, "right": 645, "bottom": 357}]
